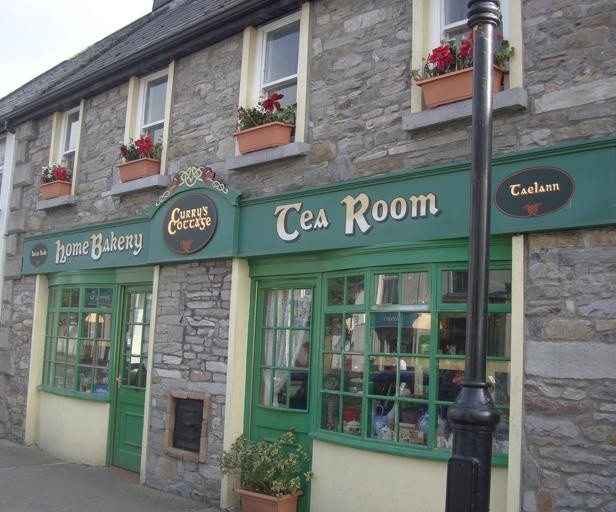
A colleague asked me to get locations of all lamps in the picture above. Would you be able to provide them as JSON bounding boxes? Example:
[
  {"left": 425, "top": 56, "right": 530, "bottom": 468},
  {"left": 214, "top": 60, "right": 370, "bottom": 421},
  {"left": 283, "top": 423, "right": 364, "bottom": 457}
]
[
  {"left": 83, "top": 313, "right": 104, "bottom": 324},
  {"left": 410, "top": 285, "right": 444, "bottom": 330}
]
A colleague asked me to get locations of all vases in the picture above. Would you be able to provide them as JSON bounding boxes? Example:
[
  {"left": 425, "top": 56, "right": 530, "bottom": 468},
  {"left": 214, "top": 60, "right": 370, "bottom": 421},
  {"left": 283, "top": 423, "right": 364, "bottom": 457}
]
[
  {"left": 414, "top": 64, "right": 505, "bottom": 111},
  {"left": 39, "top": 179, "right": 72, "bottom": 200},
  {"left": 115, "top": 158, "right": 160, "bottom": 183},
  {"left": 231, "top": 121, "right": 295, "bottom": 154}
]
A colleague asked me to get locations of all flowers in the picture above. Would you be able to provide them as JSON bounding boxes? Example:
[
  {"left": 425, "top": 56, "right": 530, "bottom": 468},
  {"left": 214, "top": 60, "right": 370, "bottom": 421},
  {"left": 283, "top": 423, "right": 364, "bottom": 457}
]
[
  {"left": 407, "top": 30, "right": 516, "bottom": 82},
  {"left": 39, "top": 164, "right": 72, "bottom": 185},
  {"left": 119, "top": 136, "right": 164, "bottom": 161},
  {"left": 233, "top": 92, "right": 297, "bottom": 132}
]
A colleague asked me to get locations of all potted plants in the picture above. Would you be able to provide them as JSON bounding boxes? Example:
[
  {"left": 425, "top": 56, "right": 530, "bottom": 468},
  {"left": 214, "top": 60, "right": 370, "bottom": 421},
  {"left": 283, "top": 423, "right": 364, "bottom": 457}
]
[{"left": 215, "top": 429, "right": 313, "bottom": 512}]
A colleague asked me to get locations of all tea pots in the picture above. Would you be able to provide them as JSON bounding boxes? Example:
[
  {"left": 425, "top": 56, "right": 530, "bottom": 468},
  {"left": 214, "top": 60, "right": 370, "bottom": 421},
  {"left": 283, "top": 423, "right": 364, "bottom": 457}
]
[{"left": 344, "top": 417, "right": 360, "bottom": 434}]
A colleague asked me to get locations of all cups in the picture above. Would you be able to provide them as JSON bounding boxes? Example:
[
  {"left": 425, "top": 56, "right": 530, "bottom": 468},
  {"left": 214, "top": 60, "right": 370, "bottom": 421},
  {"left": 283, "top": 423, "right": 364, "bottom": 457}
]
[
  {"left": 437, "top": 436, "right": 447, "bottom": 447},
  {"left": 380, "top": 424, "right": 395, "bottom": 441}
]
